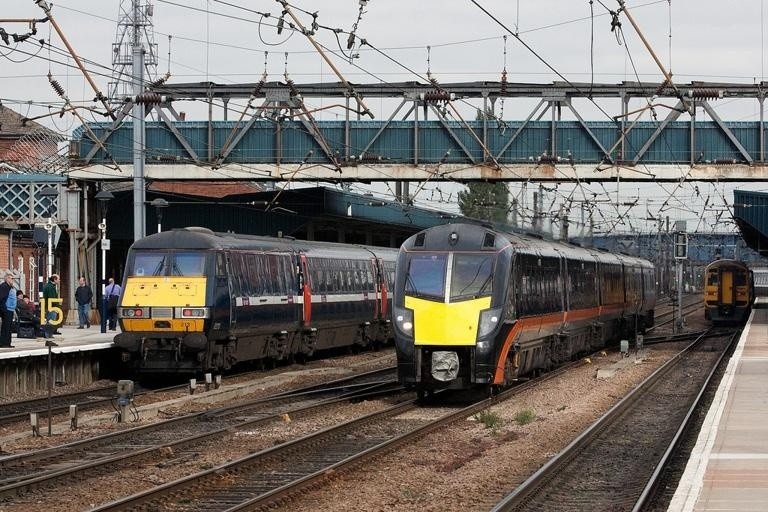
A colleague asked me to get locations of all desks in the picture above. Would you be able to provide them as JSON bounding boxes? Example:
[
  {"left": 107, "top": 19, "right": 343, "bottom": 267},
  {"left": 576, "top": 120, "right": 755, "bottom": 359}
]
[
  {"left": 39, "top": 187, "right": 61, "bottom": 279},
  {"left": 151, "top": 198, "right": 169, "bottom": 233},
  {"left": 94, "top": 191, "right": 114, "bottom": 333}
]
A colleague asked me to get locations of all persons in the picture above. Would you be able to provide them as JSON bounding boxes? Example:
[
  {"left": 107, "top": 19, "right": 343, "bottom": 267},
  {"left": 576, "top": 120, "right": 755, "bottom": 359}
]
[
  {"left": 43, "top": 274, "right": 61, "bottom": 346},
  {"left": 22, "top": 295, "right": 36, "bottom": 314},
  {"left": 105, "top": 277, "right": 122, "bottom": 330},
  {"left": 15, "top": 289, "right": 36, "bottom": 320},
  {"left": 74, "top": 277, "right": 93, "bottom": 329},
  {"left": 0, "top": 273, "right": 17, "bottom": 348}
]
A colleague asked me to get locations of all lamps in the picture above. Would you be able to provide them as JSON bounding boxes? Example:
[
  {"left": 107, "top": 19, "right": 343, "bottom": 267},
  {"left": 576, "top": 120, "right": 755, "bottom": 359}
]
[{"left": 13, "top": 306, "right": 36, "bottom": 337}]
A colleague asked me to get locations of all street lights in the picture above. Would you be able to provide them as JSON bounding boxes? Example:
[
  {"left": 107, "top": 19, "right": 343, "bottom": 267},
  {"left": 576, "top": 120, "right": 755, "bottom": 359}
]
[
  {"left": 17, "top": 326, "right": 35, "bottom": 338},
  {"left": 108, "top": 295, "right": 115, "bottom": 309}
]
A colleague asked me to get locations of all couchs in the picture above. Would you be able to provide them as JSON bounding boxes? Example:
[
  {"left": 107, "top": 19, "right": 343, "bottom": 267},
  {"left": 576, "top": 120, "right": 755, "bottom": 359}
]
[{"left": 672, "top": 232, "right": 688, "bottom": 261}]
